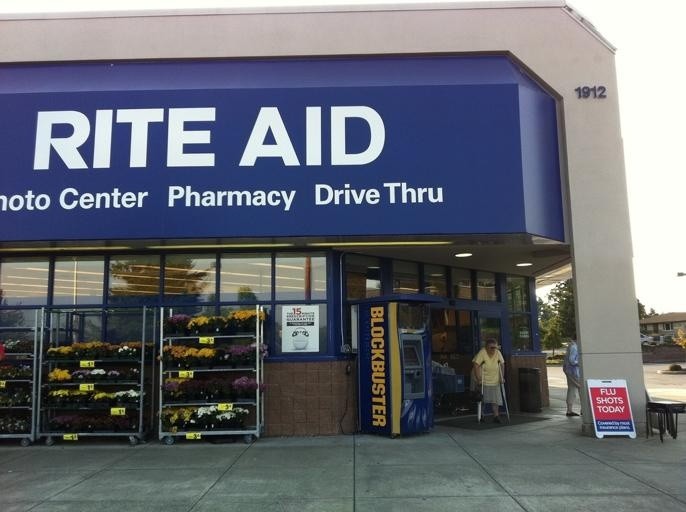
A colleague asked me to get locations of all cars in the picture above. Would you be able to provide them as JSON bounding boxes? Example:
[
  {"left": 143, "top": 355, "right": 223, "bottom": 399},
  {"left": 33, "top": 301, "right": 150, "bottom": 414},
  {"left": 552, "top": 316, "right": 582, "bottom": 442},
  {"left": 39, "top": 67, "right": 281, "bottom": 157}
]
[{"left": 640, "top": 333, "right": 653, "bottom": 346}]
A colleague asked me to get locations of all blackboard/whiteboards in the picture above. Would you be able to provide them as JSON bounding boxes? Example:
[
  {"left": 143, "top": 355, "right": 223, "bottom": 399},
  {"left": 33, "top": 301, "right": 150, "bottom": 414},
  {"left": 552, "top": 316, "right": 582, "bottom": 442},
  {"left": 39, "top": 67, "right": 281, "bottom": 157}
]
[{"left": 587, "top": 379, "right": 636, "bottom": 435}]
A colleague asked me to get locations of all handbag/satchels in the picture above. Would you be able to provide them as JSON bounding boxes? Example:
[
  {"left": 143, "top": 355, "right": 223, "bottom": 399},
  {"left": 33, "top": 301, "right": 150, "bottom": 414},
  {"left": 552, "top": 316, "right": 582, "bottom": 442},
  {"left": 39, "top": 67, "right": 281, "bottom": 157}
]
[{"left": 472, "top": 387, "right": 483, "bottom": 403}]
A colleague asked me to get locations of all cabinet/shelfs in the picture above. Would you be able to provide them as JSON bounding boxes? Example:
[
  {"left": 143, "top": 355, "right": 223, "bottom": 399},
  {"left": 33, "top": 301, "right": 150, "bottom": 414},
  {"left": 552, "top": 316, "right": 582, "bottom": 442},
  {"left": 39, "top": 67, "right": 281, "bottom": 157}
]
[{"left": 0, "top": 305, "right": 265, "bottom": 447}]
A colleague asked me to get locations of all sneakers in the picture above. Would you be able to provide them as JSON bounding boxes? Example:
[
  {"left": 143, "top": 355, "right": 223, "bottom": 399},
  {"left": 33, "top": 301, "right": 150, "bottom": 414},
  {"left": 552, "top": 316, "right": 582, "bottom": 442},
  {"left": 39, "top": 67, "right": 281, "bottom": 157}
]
[
  {"left": 492, "top": 415, "right": 504, "bottom": 423},
  {"left": 566, "top": 412, "right": 580, "bottom": 416}
]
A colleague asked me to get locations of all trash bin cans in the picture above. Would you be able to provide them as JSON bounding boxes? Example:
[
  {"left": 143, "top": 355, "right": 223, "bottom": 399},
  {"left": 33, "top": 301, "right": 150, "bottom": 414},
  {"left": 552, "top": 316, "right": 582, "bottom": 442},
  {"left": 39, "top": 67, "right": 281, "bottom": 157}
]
[{"left": 518, "top": 368, "right": 542, "bottom": 413}]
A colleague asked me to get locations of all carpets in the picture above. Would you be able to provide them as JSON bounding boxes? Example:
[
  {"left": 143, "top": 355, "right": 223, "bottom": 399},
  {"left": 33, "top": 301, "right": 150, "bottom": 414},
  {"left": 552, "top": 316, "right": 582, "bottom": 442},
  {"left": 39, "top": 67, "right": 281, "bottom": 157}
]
[{"left": 434, "top": 413, "right": 552, "bottom": 430}]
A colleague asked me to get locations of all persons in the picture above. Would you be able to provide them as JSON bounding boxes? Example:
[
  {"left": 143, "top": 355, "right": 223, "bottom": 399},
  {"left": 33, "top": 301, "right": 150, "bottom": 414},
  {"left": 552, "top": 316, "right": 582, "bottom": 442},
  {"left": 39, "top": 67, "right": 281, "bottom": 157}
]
[
  {"left": 561, "top": 330, "right": 583, "bottom": 416},
  {"left": 471, "top": 337, "right": 506, "bottom": 424}
]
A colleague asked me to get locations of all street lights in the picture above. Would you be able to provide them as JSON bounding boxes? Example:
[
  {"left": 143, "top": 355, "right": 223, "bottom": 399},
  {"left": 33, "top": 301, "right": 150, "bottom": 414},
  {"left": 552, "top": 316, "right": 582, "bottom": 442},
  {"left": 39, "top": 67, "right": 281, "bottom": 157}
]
[{"left": 677, "top": 272, "right": 686, "bottom": 277}]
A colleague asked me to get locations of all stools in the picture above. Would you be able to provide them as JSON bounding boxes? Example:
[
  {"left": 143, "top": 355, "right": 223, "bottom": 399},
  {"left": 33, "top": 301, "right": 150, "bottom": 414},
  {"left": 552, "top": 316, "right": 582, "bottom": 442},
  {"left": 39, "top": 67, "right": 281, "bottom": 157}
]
[{"left": 646, "top": 401, "right": 686, "bottom": 443}]
